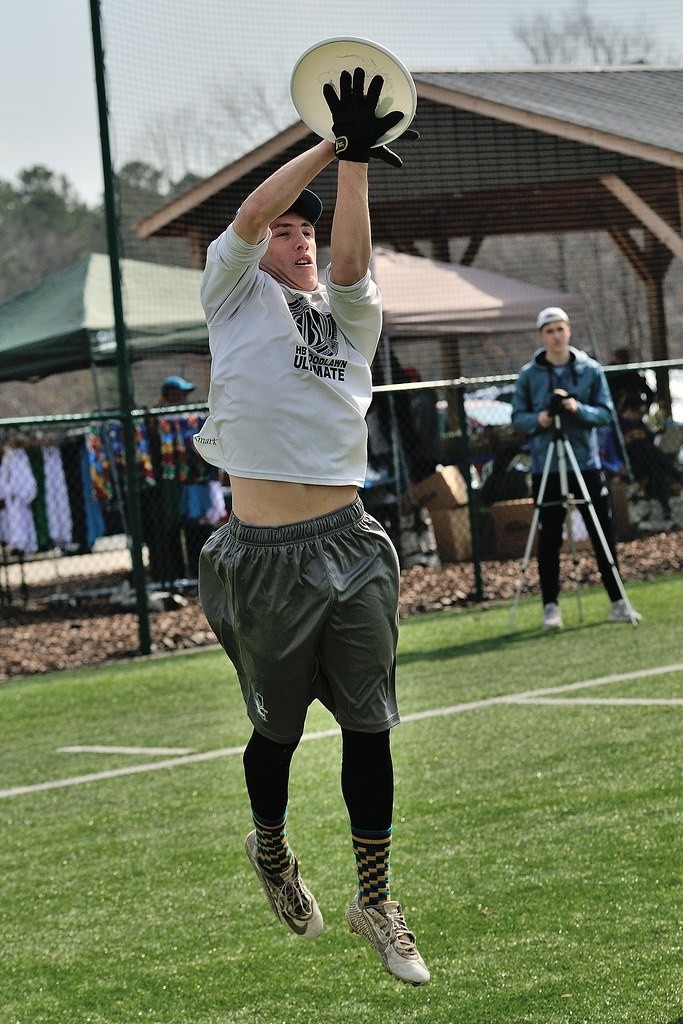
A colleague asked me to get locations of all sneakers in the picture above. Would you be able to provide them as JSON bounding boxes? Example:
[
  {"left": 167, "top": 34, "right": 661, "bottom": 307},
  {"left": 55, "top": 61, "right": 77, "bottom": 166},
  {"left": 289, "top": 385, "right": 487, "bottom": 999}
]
[
  {"left": 610, "top": 601, "right": 642, "bottom": 622},
  {"left": 544, "top": 603, "right": 564, "bottom": 630},
  {"left": 244, "top": 829, "right": 323, "bottom": 939},
  {"left": 345, "top": 893, "right": 430, "bottom": 987}
]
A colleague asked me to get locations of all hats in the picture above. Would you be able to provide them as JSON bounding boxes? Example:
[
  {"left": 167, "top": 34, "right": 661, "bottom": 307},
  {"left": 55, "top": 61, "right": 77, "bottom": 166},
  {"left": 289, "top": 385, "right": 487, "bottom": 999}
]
[
  {"left": 161, "top": 375, "right": 195, "bottom": 397},
  {"left": 280, "top": 189, "right": 323, "bottom": 226},
  {"left": 537, "top": 307, "right": 569, "bottom": 330}
]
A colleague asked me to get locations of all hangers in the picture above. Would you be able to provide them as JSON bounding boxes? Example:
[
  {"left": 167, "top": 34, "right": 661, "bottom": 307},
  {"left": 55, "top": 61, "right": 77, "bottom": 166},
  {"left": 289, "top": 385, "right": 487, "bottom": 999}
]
[{"left": 0, "top": 415, "right": 87, "bottom": 448}]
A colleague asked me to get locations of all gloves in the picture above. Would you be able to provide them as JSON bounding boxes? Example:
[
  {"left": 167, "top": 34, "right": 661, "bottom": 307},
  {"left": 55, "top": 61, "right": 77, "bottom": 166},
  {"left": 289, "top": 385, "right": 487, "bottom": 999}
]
[
  {"left": 370, "top": 129, "right": 420, "bottom": 168},
  {"left": 323, "top": 67, "right": 404, "bottom": 163}
]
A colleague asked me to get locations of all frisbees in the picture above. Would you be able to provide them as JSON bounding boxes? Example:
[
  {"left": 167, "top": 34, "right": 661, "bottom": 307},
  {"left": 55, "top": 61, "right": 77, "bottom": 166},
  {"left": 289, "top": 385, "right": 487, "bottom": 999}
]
[{"left": 290, "top": 34, "right": 419, "bottom": 150}]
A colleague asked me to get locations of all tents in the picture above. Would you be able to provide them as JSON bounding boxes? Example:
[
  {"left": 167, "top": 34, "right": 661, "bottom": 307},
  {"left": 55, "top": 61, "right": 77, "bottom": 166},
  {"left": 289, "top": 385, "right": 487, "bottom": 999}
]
[
  {"left": 1, "top": 254, "right": 210, "bottom": 542},
  {"left": 318, "top": 246, "right": 634, "bottom": 520}
]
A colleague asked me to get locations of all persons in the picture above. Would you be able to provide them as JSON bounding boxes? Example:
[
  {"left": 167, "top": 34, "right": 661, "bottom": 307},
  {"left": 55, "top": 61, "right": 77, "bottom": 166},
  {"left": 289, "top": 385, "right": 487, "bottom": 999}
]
[
  {"left": 600, "top": 349, "right": 683, "bottom": 522},
  {"left": 144, "top": 377, "right": 198, "bottom": 579},
  {"left": 404, "top": 367, "right": 439, "bottom": 454},
  {"left": 192, "top": 65, "right": 431, "bottom": 987},
  {"left": 512, "top": 307, "right": 642, "bottom": 632}
]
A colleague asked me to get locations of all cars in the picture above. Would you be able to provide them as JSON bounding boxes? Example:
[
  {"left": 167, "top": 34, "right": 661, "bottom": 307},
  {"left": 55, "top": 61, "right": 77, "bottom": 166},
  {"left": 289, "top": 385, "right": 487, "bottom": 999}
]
[{"left": 435, "top": 362, "right": 683, "bottom": 495}]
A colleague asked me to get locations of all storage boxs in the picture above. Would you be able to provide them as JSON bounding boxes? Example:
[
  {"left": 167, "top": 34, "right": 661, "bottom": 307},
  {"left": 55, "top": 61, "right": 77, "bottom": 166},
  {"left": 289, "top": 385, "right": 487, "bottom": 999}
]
[
  {"left": 430, "top": 504, "right": 475, "bottom": 563},
  {"left": 492, "top": 492, "right": 575, "bottom": 558},
  {"left": 401, "top": 465, "right": 469, "bottom": 513}
]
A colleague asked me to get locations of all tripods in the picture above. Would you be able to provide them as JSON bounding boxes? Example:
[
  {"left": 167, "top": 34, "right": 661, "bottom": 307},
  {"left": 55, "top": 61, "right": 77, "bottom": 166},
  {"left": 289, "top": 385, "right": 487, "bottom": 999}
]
[{"left": 507, "top": 412, "right": 637, "bottom": 635}]
